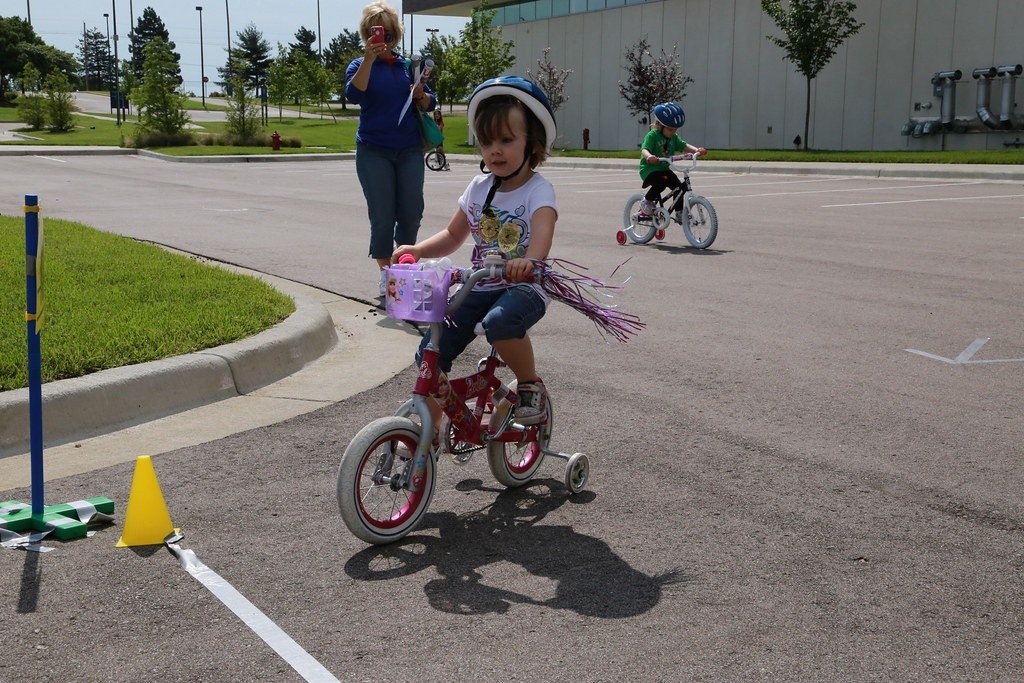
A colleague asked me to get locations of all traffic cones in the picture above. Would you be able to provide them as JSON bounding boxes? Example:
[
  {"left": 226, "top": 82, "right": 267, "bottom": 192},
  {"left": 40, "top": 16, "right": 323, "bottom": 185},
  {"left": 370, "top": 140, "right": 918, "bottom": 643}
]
[{"left": 114, "top": 453, "right": 182, "bottom": 550}]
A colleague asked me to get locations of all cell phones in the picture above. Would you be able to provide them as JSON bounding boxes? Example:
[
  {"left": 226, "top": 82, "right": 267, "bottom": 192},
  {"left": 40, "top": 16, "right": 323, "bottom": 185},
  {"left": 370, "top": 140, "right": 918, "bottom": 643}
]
[{"left": 371, "top": 26, "right": 384, "bottom": 44}]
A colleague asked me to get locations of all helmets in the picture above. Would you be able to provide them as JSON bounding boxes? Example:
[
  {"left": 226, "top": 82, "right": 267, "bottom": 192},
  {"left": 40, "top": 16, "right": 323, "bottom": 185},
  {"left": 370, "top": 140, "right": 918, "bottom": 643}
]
[
  {"left": 654, "top": 101, "right": 685, "bottom": 128},
  {"left": 466, "top": 74, "right": 557, "bottom": 158}
]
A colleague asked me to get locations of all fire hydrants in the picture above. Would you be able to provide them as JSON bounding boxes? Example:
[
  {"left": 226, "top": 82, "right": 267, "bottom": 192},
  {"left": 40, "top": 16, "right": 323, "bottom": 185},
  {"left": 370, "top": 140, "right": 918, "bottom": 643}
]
[{"left": 271, "top": 131, "right": 282, "bottom": 150}]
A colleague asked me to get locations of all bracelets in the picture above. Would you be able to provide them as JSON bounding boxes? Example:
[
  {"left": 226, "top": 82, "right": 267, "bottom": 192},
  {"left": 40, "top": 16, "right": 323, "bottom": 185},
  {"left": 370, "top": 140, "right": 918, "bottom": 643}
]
[{"left": 417, "top": 91, "right": 425, "bottom": 100}]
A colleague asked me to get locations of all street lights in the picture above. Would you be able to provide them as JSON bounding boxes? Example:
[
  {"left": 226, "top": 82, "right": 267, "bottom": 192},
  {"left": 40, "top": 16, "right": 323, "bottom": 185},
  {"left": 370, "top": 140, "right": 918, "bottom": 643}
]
[
  {"left": 425, "top": 28, "right": 439, "bottom": 55},
  {"left": 195, "top": 6, "right": 205, "bottom": 107},
  {"left": 103, "top": 13, "right": 113, "bottom": 115}
]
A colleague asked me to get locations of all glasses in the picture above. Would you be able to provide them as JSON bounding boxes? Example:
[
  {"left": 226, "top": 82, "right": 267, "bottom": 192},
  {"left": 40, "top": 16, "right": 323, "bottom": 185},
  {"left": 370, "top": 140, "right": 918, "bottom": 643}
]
[{"left": 368, "top": 32, "right": 393, "bottom": 44}]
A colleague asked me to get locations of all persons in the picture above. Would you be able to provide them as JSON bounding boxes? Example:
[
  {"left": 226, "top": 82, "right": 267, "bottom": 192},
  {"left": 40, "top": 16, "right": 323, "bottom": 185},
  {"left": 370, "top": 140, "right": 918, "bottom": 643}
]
[
  {"left": 390, "top": 76, "right": 559, "bottom": 460},
  {"left": 345, "top": 1, "right": 437, "bottom": 296},
  {"left": 639, "top": 102, "right": 708, "bottom": 224},
  {"left": 434, "top": 109, "right": 450, "bottom": 170}
]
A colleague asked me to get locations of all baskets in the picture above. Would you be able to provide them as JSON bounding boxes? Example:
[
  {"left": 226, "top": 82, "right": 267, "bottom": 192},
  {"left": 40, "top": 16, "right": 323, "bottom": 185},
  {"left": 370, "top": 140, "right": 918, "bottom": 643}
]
[{"left": 381, "top": 263, "right": 453, "bottom": 322}]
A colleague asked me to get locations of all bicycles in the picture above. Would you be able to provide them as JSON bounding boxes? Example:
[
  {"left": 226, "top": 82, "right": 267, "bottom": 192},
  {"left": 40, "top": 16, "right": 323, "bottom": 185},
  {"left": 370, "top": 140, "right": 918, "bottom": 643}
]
[
  {"left": 334, "top": 252, "right": 590, "bottom": 546},
  {"left": 615, "top": 147, "right": 721, "bottom": 250},
  {"left": 425, "top": 146, "right": 446, "bottom": 171}
]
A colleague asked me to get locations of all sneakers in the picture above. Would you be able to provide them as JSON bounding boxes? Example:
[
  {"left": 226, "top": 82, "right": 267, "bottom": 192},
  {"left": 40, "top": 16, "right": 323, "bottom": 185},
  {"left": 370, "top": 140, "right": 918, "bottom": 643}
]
[
  {"left": 675, "top": 210, "right": 690, "bottom": 224},
  {"left": 514, "top": 379, "right": 549, "bottom": 424},
  {"left": 639, "top": 196, "right": 653, "bottom": 216},
  {"left": 395, "top": 441, "right": 438, "bottom": 459}
]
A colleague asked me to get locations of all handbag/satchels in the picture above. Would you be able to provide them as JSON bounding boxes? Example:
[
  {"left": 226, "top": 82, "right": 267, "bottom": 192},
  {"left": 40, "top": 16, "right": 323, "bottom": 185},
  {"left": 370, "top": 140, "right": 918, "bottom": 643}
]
[{"left": 406, "top": 57, "right": 445, "bottom": 151}]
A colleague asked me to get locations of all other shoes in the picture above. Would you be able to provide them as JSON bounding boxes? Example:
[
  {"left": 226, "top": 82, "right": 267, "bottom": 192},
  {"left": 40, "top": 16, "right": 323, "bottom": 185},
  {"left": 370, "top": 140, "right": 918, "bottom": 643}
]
[{"left": 378, "top": 270, "right": 389, "bottom": 297}]
